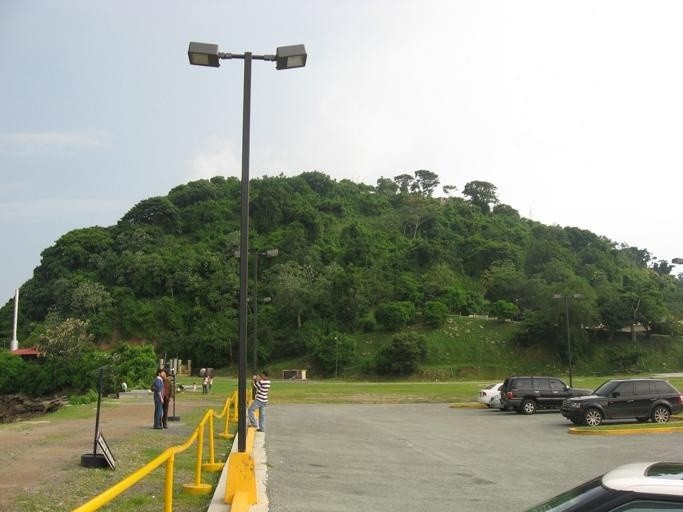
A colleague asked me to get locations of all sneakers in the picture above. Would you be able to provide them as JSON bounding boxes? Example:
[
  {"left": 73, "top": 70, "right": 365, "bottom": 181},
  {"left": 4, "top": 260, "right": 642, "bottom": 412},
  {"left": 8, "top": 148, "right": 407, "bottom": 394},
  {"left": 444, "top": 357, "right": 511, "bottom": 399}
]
[{"left": 248, "top": 423, "right": 264, "bottom": 432}]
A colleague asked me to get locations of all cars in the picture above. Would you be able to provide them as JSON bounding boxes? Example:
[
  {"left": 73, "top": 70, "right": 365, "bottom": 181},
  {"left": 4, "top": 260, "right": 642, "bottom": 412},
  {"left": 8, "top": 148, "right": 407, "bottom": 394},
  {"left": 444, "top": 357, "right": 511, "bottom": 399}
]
[
  {"left": 479, "top": 376, "right": 683, "bottom": 427},
  {"left": 524, "top": 460, "right": 683, "bottom": 512}
]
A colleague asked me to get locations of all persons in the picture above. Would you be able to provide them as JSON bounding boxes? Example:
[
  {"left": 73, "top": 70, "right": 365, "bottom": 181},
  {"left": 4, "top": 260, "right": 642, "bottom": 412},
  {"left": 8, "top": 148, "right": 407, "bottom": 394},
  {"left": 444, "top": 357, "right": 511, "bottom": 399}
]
[
  {"left": 203, "top": 368, "right": 213, "bottom": 396},
  {"left": 248, "top": 370, "right": 271, "bottom": 432},
  {"left": 153, "top": 370, "right": 172, "bottom": 429}
]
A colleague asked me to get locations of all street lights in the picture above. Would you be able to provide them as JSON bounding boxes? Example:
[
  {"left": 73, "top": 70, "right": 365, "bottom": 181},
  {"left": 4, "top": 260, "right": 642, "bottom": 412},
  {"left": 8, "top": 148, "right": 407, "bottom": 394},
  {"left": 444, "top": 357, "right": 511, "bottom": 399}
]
[
  {"left": 188, "top": 40, "right": 308, "bottom": 451},
  {"left": 552, "top": 294, "right": 584, "bottom": 388},
  {"left": 234, "top": 248, "right": 279, "bottom": 400}
]
